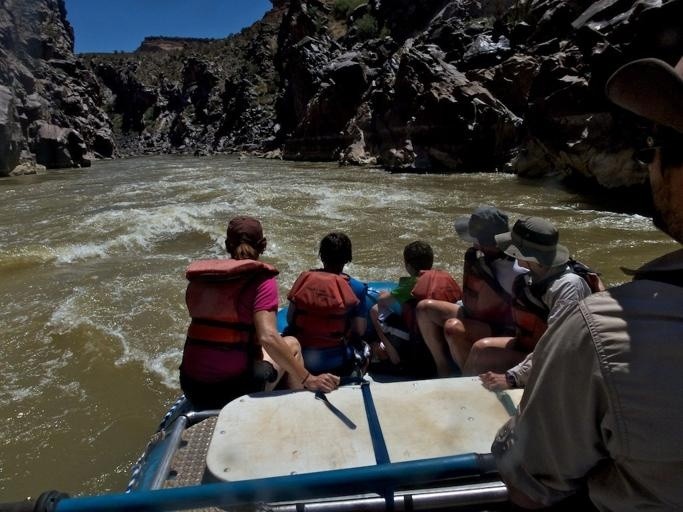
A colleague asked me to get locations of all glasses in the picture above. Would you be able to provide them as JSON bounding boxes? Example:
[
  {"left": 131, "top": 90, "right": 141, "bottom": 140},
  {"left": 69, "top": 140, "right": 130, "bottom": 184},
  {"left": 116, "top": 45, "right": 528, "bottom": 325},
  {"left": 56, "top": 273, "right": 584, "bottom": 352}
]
[{"left": 628, "top": 137, "right": 683, "bottom": 164}]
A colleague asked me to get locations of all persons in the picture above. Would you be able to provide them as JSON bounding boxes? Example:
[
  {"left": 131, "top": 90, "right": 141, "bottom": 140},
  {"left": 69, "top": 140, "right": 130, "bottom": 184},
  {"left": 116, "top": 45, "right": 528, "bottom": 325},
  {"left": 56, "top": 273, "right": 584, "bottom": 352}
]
[
  {"left": 177, "top": 214, "right": 342, "bottom": 410},
  {"left": 461, "top": 220, "right": 590, "bottom": 392},
  {"left": 414, "top": 207, "right": 529, "bottom": 376},
  {"left": 371, "top": 240, "right": 461, "bottom": 374},
  {"left": 280, "top": 232, "right": 377, "bottom": 383},
  {"left": 491, "top": 121, "right": 683, "bottom": 510}
]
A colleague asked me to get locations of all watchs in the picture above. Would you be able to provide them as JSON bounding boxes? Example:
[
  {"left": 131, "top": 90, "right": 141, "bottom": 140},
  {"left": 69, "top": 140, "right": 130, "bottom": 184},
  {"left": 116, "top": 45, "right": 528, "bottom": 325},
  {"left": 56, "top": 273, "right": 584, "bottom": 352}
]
[{"left": 505, "top": 371, "right": 516, "bottom": 388}]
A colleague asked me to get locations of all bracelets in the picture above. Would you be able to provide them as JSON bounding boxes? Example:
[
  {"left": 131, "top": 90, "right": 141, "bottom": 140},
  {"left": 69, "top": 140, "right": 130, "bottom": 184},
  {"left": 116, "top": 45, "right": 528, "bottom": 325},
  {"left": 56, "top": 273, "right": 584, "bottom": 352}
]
[{"left": 300, "top": 373, "right": 311, "bottom": 385}]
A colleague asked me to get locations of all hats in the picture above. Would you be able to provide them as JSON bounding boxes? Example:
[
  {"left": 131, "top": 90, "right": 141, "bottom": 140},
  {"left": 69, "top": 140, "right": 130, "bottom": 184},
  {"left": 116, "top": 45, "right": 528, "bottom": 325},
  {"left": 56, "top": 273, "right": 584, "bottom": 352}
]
[
  {"left": 452, "top": 204, "right": 513, "bottom": 249},
  {"left": 225, "top": 214, "right": 265, "bottom": 246},
  {"left": 493, "top": 215, "right": 577, "bottom": 266},
  {"left": 602, "top": 55, "right": 683, "bottom": 136}
]
